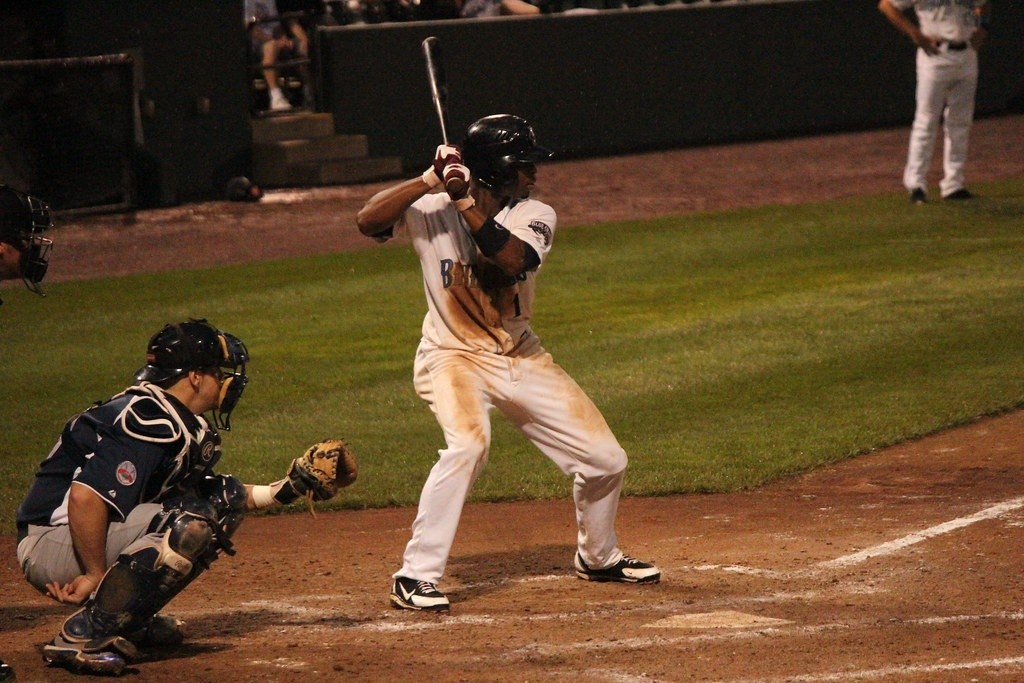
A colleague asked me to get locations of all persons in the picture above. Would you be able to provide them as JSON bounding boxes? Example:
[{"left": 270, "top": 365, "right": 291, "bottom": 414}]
[
  {"left": 16, "top": 317, "right": 359, "bottom": 676},
  {"left": 244, "top": 0, "right": 324, "bottom": 114},
  {"left": 0, "top": 182, "right": 54, "bottom": 683},
  {"left": 356, "top": 114, "right": 660, "bottom": 610},
  {"left": 878, "top": 0, "right": 992, "bottom": 205},
  {"left": 314, "top": 0, "right": 541, "bottom": 25}
]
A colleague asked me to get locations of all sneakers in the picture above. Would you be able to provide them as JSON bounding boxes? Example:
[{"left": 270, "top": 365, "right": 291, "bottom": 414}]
[
  {"left": 574, "top": 547, "right": 660, "bottom": 583},
  {"left": 389, "top": 576, "right": 450, "bottom": 613},
  {"left": 44, "top": 628, "right": 125, "bottom": 677},
  {"left": 129, "top": 619, "right": 183, "bottom": 651}
]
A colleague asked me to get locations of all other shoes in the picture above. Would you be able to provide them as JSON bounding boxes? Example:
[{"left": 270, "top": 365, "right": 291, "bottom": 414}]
[
  {"left": 910, "top": 188, "right": 928, "bottom": 206},
  {"left": 271, "top": 92, "right": 293, "bottom": 110},
  {"left": 945, "top": 189, "right": 971, "bottom": 200}
]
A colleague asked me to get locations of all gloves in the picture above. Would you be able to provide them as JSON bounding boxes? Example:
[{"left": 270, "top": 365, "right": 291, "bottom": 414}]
[
  {"left": 422, "top": 143, "right": 464, "bottom": 188},
  {"left": 442, "top": 157, "right": 475, "bottom": 212}
]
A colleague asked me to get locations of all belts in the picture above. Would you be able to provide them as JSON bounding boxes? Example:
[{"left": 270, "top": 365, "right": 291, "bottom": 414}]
[{"left": 936, "top": 40, "right": 967, "bottom": 50}]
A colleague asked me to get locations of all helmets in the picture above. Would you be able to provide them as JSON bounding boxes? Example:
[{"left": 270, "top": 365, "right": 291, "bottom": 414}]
[
  {"left": 0, "top": 185, "right": 34, "bottom": 250},
  {"left": 465, "top": 114, "right": 553, "bottom": 190},
  {"left": 133, "top": 316, "right": 223, "bottom": 382}
]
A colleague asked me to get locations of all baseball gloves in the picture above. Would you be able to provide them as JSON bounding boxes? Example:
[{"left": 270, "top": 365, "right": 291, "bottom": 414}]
[{"left": 284, "top": 438, "right": 359, "bottom": 500}]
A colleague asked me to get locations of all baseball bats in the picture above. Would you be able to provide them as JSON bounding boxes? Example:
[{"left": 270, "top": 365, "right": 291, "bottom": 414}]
[{"left": 421, "top": 35, "right": 464, "bottom": 194}]
